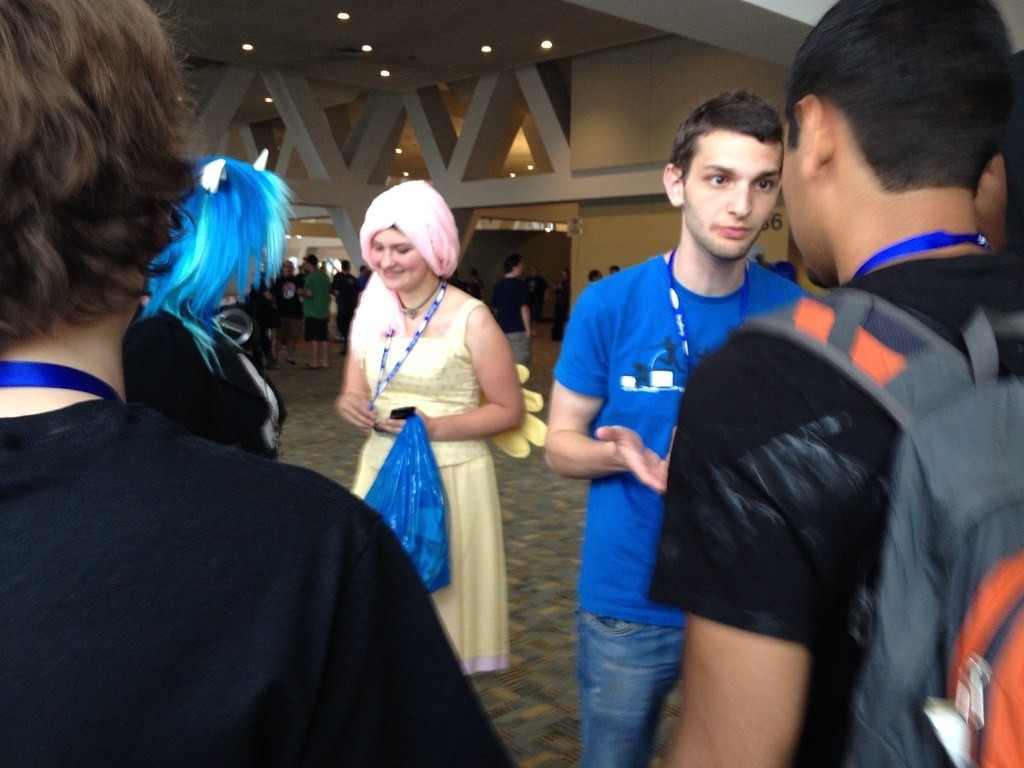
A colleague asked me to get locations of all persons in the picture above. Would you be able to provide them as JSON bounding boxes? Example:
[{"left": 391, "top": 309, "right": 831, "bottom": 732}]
[
  {"left": 543, "top": 87, "right": 815, "bottom": 767},
  {"left": 0, "top": 0, "right": 521, "bottom": 768},
  {"left": 333, "top": 180, "right": 525, "bottom": 676},
  {"left": 246, "top": 253, "right": 622, "bottom": 364},
  {"left": 642, "top": 1, "right": 1024, "bottom": 767},
  {"left": 121, "top": 148, "right": 296, "bottom": 464}
]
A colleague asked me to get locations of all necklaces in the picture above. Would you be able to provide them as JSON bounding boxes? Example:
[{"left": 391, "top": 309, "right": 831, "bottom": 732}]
[{"left": 397, "top": 280, "right": 441, "bottom": 319}]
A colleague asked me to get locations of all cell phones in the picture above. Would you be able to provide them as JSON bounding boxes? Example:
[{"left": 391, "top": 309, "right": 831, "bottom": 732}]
[{"left": 391, "top": 407, "right": 415, "bottom": 420}]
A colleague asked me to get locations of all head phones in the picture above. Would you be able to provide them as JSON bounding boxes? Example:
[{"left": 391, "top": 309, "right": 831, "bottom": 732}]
[{"left": 180, "top": 301, "right": 260, "bottom": 350}]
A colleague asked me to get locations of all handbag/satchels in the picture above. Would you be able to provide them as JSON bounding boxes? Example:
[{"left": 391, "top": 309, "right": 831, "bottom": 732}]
[{"left": 364, "top": 414, "right": 452, "bottom": 596}]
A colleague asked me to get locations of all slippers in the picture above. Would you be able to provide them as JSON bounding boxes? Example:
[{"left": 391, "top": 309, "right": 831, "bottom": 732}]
[
  {"left": 302, "top": 364, "right": 318, "bottom": 370},
  {"left": 319, "top": 362, "right": 331, "bottom": 369}
]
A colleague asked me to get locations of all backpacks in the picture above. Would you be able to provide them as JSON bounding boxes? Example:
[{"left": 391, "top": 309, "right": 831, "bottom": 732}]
[{"left": 742, "top": 284, "right": 1024, "bottom": 768}]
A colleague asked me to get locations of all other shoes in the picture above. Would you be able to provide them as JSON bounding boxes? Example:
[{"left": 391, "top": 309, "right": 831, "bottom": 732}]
[{"left": 286, "top": 357, "right": 296, "bottom": 366}]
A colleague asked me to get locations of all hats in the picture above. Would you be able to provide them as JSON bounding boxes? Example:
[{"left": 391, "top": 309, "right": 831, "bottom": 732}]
[{"left": 303, "top": 254, "right": 319, "bottom": 269}]
